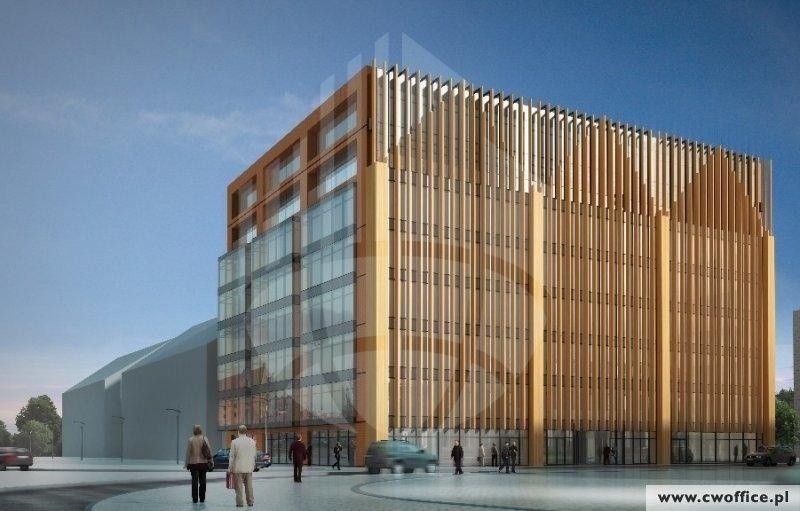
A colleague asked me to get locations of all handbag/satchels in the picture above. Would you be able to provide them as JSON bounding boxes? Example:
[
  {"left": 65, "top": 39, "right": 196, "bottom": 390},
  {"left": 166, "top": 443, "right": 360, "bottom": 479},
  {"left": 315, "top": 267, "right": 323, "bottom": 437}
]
[
  {"left": 226, "top": 472, "right": 235, "bottom": 489},
  {"left": 201, "top": 437, "right": 211, "bottom": 460}
]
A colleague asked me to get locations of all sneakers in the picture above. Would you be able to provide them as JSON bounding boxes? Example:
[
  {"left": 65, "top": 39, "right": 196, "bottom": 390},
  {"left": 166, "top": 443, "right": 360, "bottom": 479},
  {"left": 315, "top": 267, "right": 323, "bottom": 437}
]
[
  {"left": 452, "top": 465, "right": 517, "bottom": 474},
  {"left": 294, "top": 479, "right": 303, "bottom": 482},
  {"left": 332, "top": 465, "right": 341, "bottom": 470},
  {"left": 192, "top": 499, "right": 206, "bottom": 503},
  {"left": 236, "top": 503, "right": 254, "bottom": 507}
]
